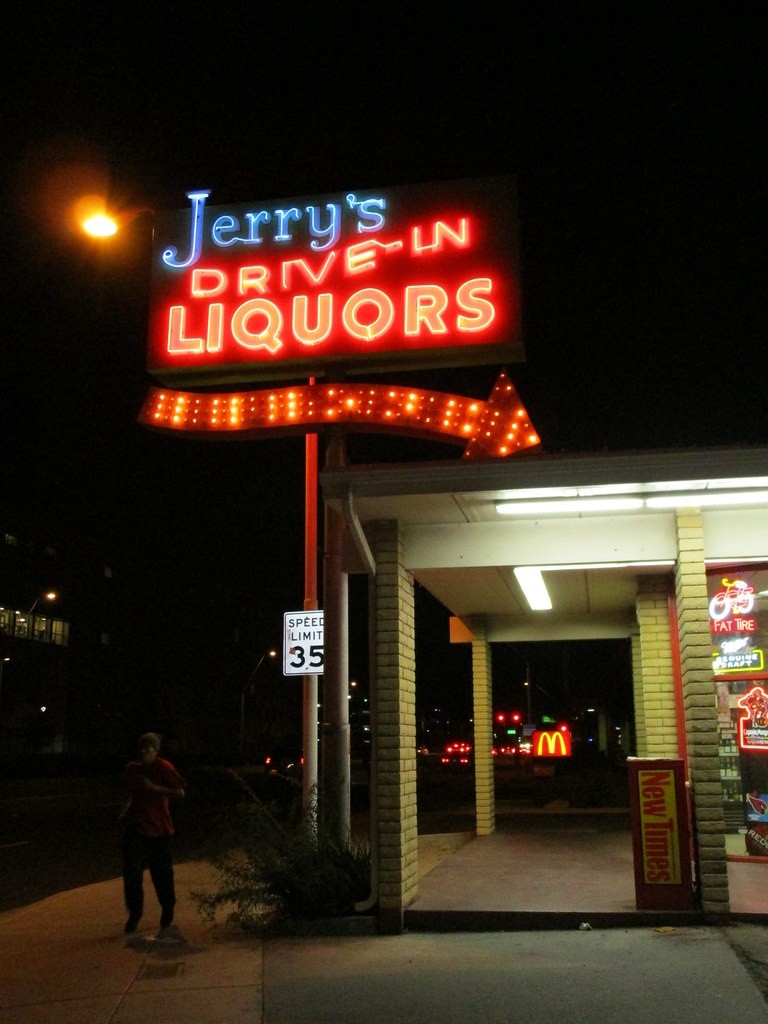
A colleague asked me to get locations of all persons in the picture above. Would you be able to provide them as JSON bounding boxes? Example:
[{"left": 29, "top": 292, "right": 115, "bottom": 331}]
[{"left": 118, "top": 733, "right": 187, "bottom": 934}]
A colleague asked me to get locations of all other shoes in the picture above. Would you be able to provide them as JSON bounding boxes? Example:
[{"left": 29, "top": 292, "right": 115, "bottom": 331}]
[
  {"left": 160, "top": 903, "right": 174, "bottom": 928},
  {"left": 125, "top": 909, "right": 143, "bottom": 932}
]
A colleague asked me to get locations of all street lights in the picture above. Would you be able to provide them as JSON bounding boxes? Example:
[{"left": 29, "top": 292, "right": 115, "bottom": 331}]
[
  {"left": 24, "top": 590, "right": 56, "bottom": 640},
  {"left": 240, "top": 649, "right": 277, "bottom": 755}
]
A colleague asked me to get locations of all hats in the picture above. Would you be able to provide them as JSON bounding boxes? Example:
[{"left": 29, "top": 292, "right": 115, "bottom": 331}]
[{"left": 139, "top": 733, "right": 160, "bottom": 753}]
